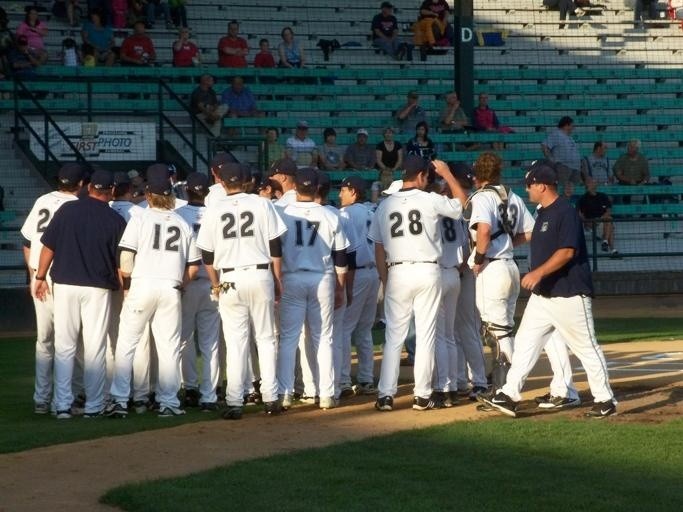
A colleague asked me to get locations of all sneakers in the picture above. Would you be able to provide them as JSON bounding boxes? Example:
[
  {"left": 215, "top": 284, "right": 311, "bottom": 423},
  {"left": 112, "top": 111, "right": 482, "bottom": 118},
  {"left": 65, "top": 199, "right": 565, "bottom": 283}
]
[
  {"left": 82, "top": 412, "right": 99, "bottom": 418},
  {"left": 475, "top": 391, "right": 516, "bottom": 417},
  {"left": 535, "top": 393, "right": 552, "bottom": 404},
  {"left": 351, "top": 383, "right": 375, "bottom": 394},
  {"left": 476, "top": 401, "right": 519, "bottom": 411},
  {"left": 263, "top": 401, "right": 276, "bottom": 415},
  {"left": 220, "top": 406, "right": 241, "bottom": 419},
  {"left": 199, "top": 402, "right": 219, "bottom": 413},
  {"left": 99, "top": 398, "right": 128, "bottom": 416},
  {"left": 584, "top": 396, "right": 617, "bottom": 419},
  {"left": 574, "top": 9, "right": 585, "bottom": 18},
  {"left": 374, "top": 396, "right": 393, "bottom": 411},
  {"left": 608, "top": 251, "right": 624, "bottom": 258},
  {"left": 434, "top": 392, "right": 453, "bottom": 407},
  {"left": 339, "top": 383, "right": 353, "bottom": 397},
  {"left": 451, "top": 391, "right": 459, "bottom": 405},
  {"left": 298, "top": 394, "right": 318, "bottom": 403},
  {"left": 412, "top": 394, "right": 439, "bottom": 410},
  {"left": 157, "top": 404, "right": 186, "bottom": 417},
  {"left": 184, "top": 389, "right": 198, "bottom": 405},
  {"left": 244, "top": 394, "right": 255, "bottom": 406},
  {"left": 55, "top": 409, "right": 72, "bottom": 419},
  {"left": 279, "top": 400, "right": 291, "bottom": 411},
  {"left": 133, "top": 398, "right": 155, "bottom": 414},
  {"left": 538, "top": 399, "right": 581, "bottom": 409},
  {"left": 215, "top": 387, "right": 226, "bottom": 402},
  {"left": 252, "top": 391, "right": 260, "bottom": 403},
  {"left": 468, "top": 386, "right": 486, "bottom": 400},
  {"left": 318, "top": 396, "right": 336, "bottom": 408},
  {"left": 601, "top": 242, "right": 608, "bottom": 251},
  {"left": 33, "top": 402, "right": 48, "bottom": 414}
]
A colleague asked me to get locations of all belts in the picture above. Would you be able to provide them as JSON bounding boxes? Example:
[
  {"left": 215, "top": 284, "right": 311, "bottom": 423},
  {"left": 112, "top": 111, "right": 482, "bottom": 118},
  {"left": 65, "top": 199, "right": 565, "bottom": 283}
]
[
  {"left": 222, "top": 264, "right": 268, "bottom": 273},
  {"left": 488, "top": 258, "right": 500, "bottom": 262},
  {"left": 354, "top": 266, "right": 364, "bottom": 268},
  {"left": 386, "top": 261, "right": 437, "bottom": 268}
]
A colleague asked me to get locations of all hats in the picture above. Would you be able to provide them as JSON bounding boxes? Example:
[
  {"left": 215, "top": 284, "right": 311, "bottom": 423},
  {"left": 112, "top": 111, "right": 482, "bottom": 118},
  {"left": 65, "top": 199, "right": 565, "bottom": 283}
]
[
  {"left": 527, "top": 158, "right": 556, "bottom": 172},
  {"left": 297, "top": 120, "right": 310, "bottom": 129},
  {"left": 218, "top": 163, "right": 241, "bottom": 183},
  {"left": 294, "top": 167, "right": 317, "bottom": 186},
  {"left": 405, "top": 155, "right": 431, "bottom": 172},
  {"left": 270, "top": 158, "right": 296, "bottom": 176},
  {"left": 90, "top": 169, "right": 113, "bottom": 189},
  {"left": 52, "top": 165, "right": 83, "bottom": 185},
  {"left": 406, "top": 90, "right": 419, "bottom": 99},
  {"left": 381, "top": 1, "right": 395, "bottom": 8},
  {"left": 356, "top": 128, "right": 368, "bottom": 136},
  {"left": 340, "top": 175, "right": 364, "bottom": 187},
  {"left": 114, "top": 171, "right": 129, "bottom": 184},
  {"left": 145, "top": 176, "right": 172, "bottom": 196},
  {"left": 315, "top": 172, "right": 330, "bottom": 186},
  {"left": 146, "top": 163, "right": 168, "bottom": 177},
  {"left": 448, "top": 162, "right": 473, "bottom": 184},
  {"left": 525, "top": 165, "right": 558, "bottom": 184},
  {"left": 186, "top": 171, "right": 207, "bottom": 186},
  {"left": 210, "top": 153, "right": 236, "bottom": 168},
  {"left": 380, "top": 179, "right": 403, "bottom": 196}
]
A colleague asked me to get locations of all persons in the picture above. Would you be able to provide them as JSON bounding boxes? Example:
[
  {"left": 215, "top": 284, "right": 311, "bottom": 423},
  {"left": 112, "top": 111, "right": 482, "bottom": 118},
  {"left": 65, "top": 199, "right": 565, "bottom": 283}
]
[
  {"left": 20, "top": 157, "right": 618, "bottom": 419},
  {"left": 1, "top": 1, "right": 674, "bottom": 256}
]
